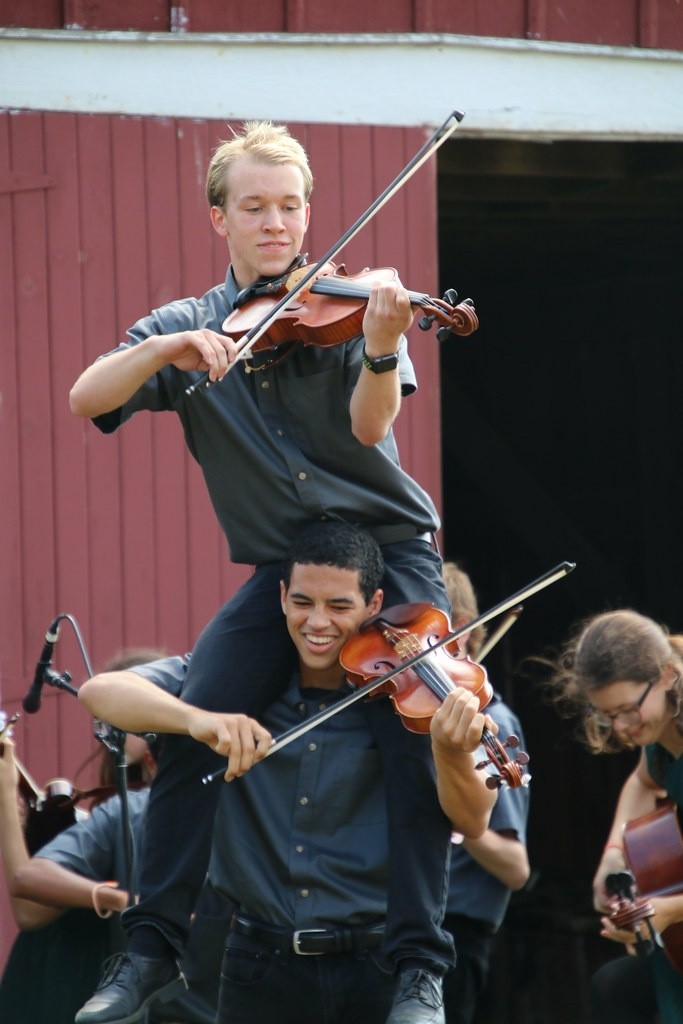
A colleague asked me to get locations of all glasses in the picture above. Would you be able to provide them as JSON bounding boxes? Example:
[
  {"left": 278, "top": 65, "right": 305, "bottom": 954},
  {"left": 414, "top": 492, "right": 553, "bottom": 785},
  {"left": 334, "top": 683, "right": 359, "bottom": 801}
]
[{"left": 587, "top": 680, "right": 654, "bottom": 728}]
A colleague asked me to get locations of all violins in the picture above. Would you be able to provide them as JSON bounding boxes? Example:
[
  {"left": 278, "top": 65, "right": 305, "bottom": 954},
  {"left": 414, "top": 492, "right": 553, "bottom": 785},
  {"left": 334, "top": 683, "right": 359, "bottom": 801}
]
[
  {"left": 338, "top": 599, "right": 535, "bottom": 791},
  {"left": 221, "top": 260, "right": 479, "bottom": 353}
]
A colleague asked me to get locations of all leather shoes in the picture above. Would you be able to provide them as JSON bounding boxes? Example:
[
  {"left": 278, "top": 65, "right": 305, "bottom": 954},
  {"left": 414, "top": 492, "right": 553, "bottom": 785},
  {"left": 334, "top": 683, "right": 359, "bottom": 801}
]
[
  {"left": 75, "top": 953, "right": 188, "bottom": 1024},
  {"left": 389, "top": 967, "right": 445, "bottom": 1024}
]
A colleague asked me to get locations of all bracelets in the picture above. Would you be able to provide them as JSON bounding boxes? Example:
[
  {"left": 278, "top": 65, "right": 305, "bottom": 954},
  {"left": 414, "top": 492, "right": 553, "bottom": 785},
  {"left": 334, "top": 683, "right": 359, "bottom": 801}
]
[
  {"left": 604, "top": 842, "right": 624, "bottom": 849},
  {"left": 89, "top": 879, "right": 120, "bottom": 920}
]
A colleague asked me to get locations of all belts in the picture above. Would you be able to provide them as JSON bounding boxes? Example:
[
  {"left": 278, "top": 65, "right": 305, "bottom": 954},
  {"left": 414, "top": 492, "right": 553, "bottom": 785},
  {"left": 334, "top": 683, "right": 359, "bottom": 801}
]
[
  {"left": 365, "top": 521, "right": 432, "bottom": 546},
  {"left": 230, "top": 915, "right": 389, "bottom": 955}
]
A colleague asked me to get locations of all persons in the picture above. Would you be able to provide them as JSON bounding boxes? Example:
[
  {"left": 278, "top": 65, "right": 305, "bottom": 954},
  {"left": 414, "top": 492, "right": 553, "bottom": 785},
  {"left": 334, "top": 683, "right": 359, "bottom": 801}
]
[
  {"left": 75, "top": 515, "right": 499, "bottom": 1024},
  {"left": 441, "top": 560, "right": 533, "bottom": 1024},
  {"left": 518, "top": 606, "right": 683, "bottom": 1024},
  {"left": 64, "top": 119, "right": 511, "bottom": 1024},
  {"left": 0, "top": 645, "right": 187, "bottom": 1024}
]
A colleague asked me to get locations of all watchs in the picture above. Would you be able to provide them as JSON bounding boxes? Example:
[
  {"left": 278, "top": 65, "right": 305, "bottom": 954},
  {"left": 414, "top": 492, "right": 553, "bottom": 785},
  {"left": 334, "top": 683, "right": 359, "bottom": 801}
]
[{"left": 360, "top": 342, "right": 402, "bottom": 376}]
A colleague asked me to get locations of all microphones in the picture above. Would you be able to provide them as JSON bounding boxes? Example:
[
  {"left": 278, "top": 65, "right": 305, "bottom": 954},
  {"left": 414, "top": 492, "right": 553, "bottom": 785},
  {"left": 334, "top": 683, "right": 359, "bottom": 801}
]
[{"left": 23, "top": 623, "right": 59, "bottom": 714}]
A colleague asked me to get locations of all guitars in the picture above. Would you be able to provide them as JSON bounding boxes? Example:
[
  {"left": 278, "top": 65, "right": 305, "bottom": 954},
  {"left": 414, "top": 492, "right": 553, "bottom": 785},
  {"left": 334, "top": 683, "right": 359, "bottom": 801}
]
[
  {"left": 604, "top": 795, "right": 682, "bottom": 976},
  {"left": 0, "top": 709, "right": 90, "bottom": 857}
]
